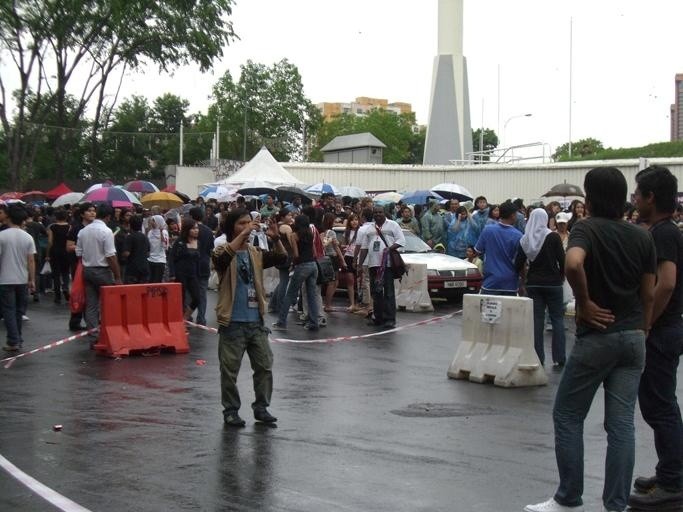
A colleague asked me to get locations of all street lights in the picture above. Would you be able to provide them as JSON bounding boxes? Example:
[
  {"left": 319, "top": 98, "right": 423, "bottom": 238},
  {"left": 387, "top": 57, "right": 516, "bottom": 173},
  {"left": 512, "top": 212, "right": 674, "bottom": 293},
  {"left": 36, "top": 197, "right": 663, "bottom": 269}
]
[{"left": 501, "top": 112, "right": 533, "bottom": 146}]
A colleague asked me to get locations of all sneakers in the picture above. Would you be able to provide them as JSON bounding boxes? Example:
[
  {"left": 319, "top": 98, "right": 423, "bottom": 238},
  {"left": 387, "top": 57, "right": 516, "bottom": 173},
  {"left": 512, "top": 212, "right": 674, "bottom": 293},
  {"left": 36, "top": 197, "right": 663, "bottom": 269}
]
[
  {"left": 22, "top": 315, "right": 29, "bottom": 321},
  {"left": 523, "top": 497, "right": 584, "bottom": 512},
  {"left": 254, "top": 411, "right": 277, "bottom": 422},
  {"left": 224, "top": 415, "right": 245, "bottom": 426},
  {"left": 627, "top": 476, "right": 683, "bottom": 512},
  {"left": 2, "top": 343, "right": 23, "bottom": 351}
]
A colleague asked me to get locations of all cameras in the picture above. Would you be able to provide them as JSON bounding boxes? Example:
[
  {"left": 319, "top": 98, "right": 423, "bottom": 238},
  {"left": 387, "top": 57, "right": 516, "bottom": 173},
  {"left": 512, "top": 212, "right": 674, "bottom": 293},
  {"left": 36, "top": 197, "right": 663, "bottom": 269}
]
[{"left": 256, "top": 222, "right": 269, "bottom": 232}]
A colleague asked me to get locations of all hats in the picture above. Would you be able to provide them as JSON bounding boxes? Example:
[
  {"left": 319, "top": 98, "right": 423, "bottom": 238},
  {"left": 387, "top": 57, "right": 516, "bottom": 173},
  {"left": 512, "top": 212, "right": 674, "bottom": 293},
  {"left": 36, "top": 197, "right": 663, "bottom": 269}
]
[{"left": 555, "top": 212, "right": 569, "bottom": 224}]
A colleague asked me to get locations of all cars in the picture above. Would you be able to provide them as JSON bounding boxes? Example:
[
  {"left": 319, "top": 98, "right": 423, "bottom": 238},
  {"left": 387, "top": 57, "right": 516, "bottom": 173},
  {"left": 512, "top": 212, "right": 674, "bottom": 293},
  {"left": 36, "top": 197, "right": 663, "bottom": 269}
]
[{"left": 325, "top": 225, "right": 481, "bottom": 302}]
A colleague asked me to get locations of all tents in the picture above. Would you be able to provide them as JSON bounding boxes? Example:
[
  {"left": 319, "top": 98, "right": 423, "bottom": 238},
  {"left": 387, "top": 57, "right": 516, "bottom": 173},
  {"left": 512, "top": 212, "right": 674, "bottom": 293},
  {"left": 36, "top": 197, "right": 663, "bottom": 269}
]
[{"left": 199, "top": 144, "right": 307, "bottom": 186}]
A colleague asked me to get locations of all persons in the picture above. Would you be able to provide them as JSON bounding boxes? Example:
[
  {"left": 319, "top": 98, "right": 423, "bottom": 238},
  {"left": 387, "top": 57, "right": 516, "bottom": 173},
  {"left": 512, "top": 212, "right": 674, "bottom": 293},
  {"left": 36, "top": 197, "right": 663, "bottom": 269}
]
[
  {"left": 621, "top": 199, "right": 682, "bottom": 231},
  {"left": 524, "top": 168, "right": 657, "bottom": 512},
  {"left": 1, "top": 194, "right": 591, "bottom": 426},
  {"left": 626, "top": 167, "right": 682, "bottom": 512}
]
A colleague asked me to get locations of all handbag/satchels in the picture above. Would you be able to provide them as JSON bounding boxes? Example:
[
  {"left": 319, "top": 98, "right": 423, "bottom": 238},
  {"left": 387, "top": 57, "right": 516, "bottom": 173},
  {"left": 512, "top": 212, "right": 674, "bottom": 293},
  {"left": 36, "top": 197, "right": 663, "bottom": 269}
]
[
  {"left": 390, "top": 250, "right": 406, "bottom": 279},
  {"left": 316, "top": 256, "right": 335, "bottom": 285}
]
